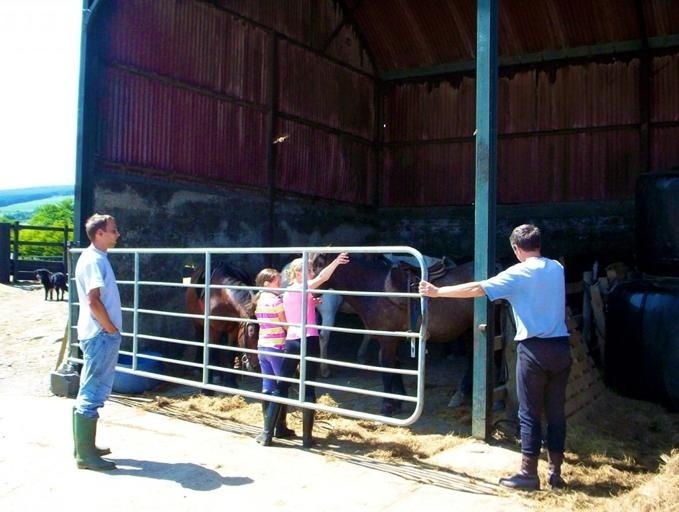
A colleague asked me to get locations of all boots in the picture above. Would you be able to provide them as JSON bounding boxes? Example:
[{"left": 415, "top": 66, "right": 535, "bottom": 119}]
[
  {"left": 499, "top": 455, "right": 540, "bottom": 489},
  {"left": 255, "top": 389, "right": 294, "bottom": 445},
  {"left": 547, "top": 452, "right": 562, "bottom": 486},
  {"left": 303, "top": 409, "right": 317, "bottom": 448},
  {"left": 75, "top": 416, "right": 115, "bottom": 470},
  {"left": 73, "top": 408, "right": 110, "bottom": 458}
]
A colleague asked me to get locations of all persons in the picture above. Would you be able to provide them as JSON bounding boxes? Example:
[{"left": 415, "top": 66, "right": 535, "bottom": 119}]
[
  {"left": 254, "top": 250, "right": 351, "bottom": 448},
  {"left": 245, "top": 266, "right": 297, "bottom": 440},
  {"left": 418, "top": 222, "right": 576, "bottom": 494},
  {"left": 68, "top": 208, "right": 123, "bottom": 471}
]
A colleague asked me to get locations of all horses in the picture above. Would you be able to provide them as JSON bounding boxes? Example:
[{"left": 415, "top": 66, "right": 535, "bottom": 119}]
[
  {"left": 185, "top": 260, "right": 260, "bottom": 397},
  {"left": 34, "top": 269, "right": 68, "bottom": 301},
  {"left": 279, "top": 252, "right": 458, "bottom": 379},
  {"left": 310, "top": 242, "right": 515, "bottom": 417}
]
